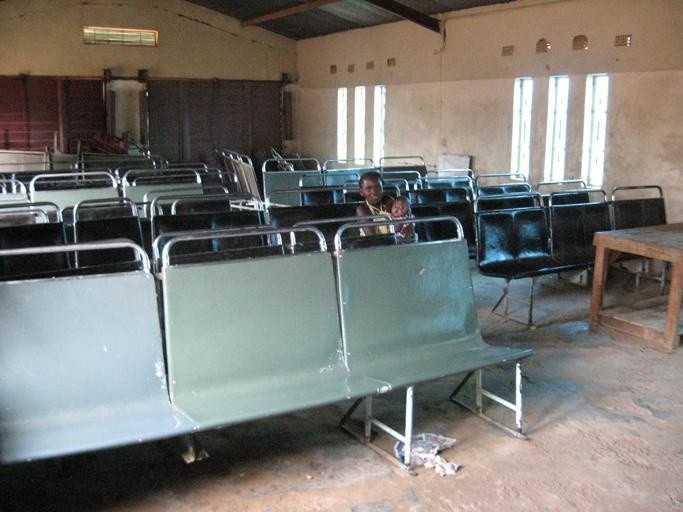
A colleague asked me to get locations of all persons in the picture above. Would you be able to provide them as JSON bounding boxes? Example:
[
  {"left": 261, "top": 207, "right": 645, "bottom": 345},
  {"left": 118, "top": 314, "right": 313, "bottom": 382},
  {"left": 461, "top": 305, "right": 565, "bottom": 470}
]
[
  {"left": 390, "top": 196, "right": 412, "bottom": 244},
  {"left": 356, "top": 171, "right": 395, "bottom": 246}
]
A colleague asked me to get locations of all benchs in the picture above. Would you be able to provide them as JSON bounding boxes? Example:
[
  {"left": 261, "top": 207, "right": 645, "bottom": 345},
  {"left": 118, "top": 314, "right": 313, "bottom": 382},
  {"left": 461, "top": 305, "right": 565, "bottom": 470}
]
[
  {"left": 0, "top": 153, "right": 527, "bottom": 476},
  {"left": 215, "top": 149, "right": 670, "bottom": 328}
]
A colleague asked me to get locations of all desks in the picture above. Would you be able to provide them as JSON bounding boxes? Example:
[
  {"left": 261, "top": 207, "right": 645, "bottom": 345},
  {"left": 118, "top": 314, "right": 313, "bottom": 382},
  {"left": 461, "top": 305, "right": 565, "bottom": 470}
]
[{"left": 582, "top": 219, "right": 682, "bottom": 353}]
